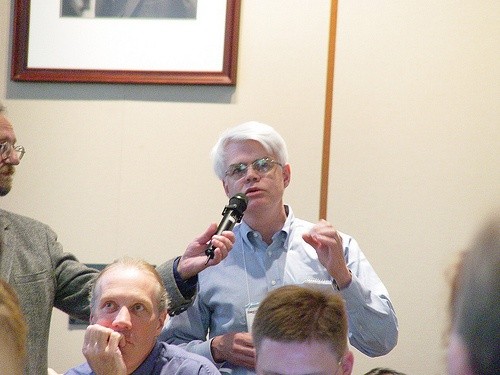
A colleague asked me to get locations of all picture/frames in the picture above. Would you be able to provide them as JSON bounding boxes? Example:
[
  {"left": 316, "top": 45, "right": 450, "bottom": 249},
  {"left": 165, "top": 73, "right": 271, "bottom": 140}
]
[{"left": 10, "top": 0, "right": 242, "bottom": 87}]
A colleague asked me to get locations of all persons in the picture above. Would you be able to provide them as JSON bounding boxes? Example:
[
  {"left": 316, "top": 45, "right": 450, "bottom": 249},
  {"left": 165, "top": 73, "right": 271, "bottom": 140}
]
[
  {"left": 62, "top": 255, "right": 222, "bottom": 374},
  {"left": 154, "top": 119, "right": 400, "bottom": 375},
  {"left": 247, "top": 281, "right": 357, "bottom": 375},
  {"left": 440, "top": 202, "right": 500, "bottom": 375},
  {"left": 0, "top": 99, "right": 238, "bottom": 375}
]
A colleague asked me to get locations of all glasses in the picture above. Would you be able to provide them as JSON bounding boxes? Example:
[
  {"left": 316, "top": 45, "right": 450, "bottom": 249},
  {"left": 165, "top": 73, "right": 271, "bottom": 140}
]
[
  {"left": 0, "top": 142, "right": 25, "bottom": 162},
  {"left": 223, "top": 156, "right": 286, "bottom": 179}
]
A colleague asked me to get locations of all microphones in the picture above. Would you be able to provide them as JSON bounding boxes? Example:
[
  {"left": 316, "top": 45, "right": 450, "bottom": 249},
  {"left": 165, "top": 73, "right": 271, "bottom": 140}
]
[{"left": 205, "top": 193, "right": 248, "bottom": 261}]
